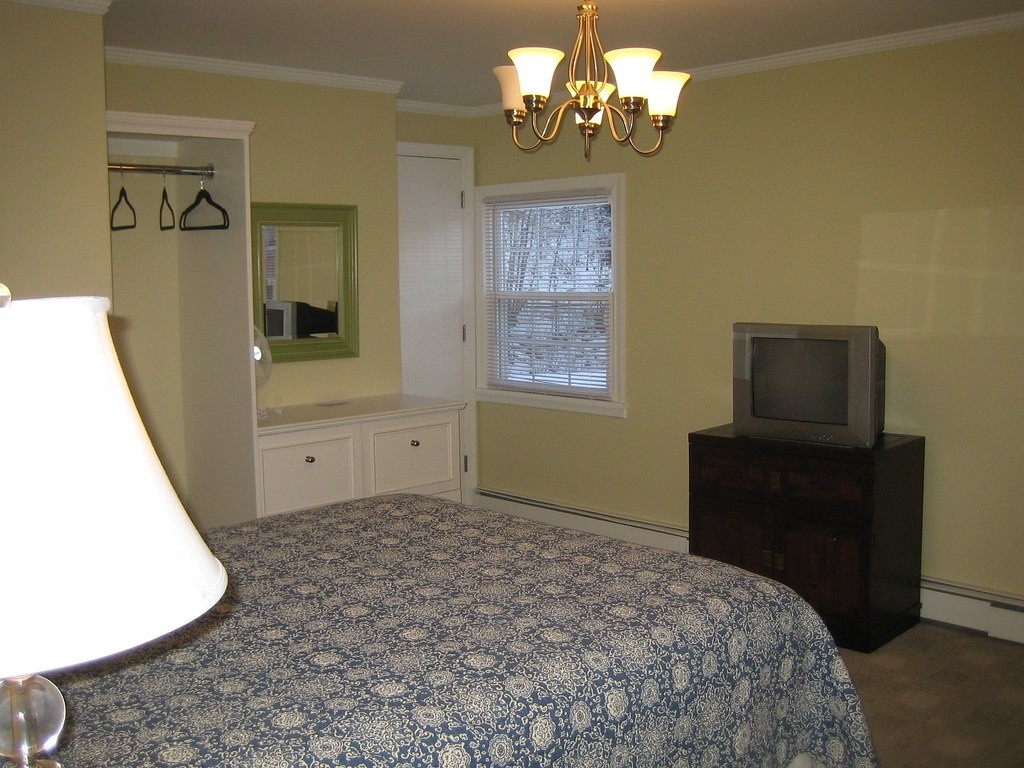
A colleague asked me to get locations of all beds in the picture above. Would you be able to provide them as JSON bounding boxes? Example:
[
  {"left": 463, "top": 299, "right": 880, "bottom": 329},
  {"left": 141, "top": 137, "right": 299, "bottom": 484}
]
[{"left": 0, "top": 492, "right": 878, "bottom": 768}]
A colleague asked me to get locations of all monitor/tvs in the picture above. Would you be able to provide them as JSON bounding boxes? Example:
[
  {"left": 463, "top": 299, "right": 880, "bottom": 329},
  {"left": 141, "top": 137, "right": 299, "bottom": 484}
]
[
  {"left": 265, "top": 302, "right": 323, "bottom": 341},
  {"left": 732, "top": 322, "right": 886, "bottom": 449}
]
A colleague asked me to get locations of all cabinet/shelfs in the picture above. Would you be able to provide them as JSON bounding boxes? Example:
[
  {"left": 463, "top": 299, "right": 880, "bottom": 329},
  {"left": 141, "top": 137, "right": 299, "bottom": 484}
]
[
  {"left": 257, "top": 394, "right": 468, "bottom": 519},
  {"left": 688, "top": 422, "right": 925, "bottom": 654}
]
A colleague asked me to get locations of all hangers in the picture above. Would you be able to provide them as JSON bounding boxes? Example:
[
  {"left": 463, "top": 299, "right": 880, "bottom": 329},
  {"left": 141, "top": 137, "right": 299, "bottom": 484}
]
[
  {"left": 179, "top": 166, "right": 230, "bottom": 231},
  {"left": 111, "top": 163, "right": 136, "bottom": 231},
  {"left": 159, "top": 165, "right": 175, "bottom": 230}
]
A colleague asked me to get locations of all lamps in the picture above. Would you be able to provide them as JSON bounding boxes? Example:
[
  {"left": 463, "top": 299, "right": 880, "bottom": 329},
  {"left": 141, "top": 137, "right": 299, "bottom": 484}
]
[
  {"left": 0, "top": 295, "right": 230, "bottom": 768},
  {"left": 494, "top": 0, "right": 691, "bottom": 159}
]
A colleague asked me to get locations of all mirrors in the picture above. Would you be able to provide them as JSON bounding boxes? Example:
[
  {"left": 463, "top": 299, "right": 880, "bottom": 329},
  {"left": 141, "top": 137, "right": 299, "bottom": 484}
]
[{"left": 251, "top": 202, "right": 360, "bottom": 363}]
